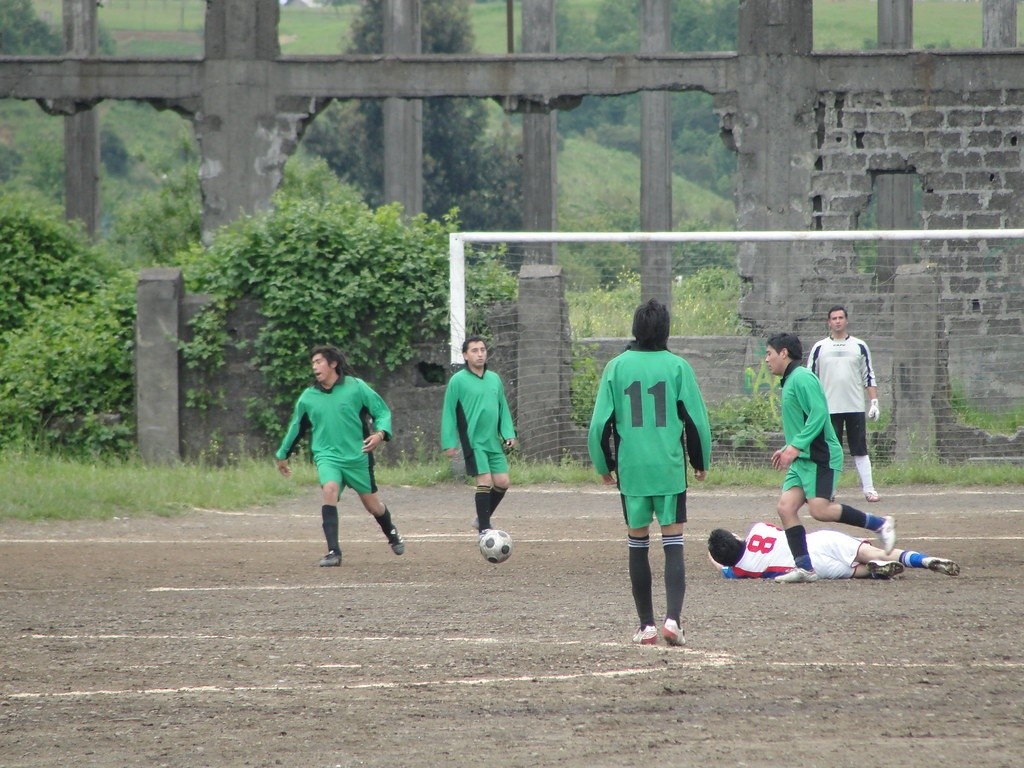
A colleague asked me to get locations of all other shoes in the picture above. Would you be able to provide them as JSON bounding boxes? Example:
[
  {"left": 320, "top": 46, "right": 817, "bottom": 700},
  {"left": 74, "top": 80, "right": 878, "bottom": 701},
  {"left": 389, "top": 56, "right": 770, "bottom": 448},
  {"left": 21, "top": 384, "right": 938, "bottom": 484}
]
[
  {"left": 866, "top": 560, "right": 905, "bottom": 578},
  {"left": 479, "top": 529, "right": 491, "bottom": 544},
  {"left": 922, "top": 557, "right": 960, "bottom": 576},
  {"left": 472, "top": 516, "right": 493, "bottom": 531}
]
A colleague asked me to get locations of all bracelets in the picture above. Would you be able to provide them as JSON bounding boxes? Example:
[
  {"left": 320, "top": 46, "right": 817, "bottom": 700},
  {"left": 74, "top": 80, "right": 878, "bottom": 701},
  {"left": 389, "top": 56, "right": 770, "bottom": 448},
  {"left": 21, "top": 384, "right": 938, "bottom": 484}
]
[{"left": 376, "top": 430, "right": 384, "bottom": 441}]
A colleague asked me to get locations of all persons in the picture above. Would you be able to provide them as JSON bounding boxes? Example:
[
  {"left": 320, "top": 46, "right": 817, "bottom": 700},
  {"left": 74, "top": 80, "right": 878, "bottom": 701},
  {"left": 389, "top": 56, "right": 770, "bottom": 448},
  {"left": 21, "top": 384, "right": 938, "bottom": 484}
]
[
  {"left": 806, "top": 306, "right": 881, "bottom": 502},
  {"left": 441, "top": 337, "right": 518, "bottom": 543},
  {"left": 587, "top": 296, "right": 711, "bottom": 645},
  {"left": 707, "top": 523, "right": 961, "bottom": 578},
  {"left": 276, "top": 343, "right": 406, "bottom": 566},
  {"left": 765, "top": 333, "right": 899, "bottom": 587}
]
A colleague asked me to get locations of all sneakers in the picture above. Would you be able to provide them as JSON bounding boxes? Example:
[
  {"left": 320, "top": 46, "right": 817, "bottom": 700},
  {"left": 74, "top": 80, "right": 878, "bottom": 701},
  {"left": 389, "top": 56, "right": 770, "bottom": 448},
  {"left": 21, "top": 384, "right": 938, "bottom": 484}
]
[
  {"left": 874, "top": 516, "right": 897, "bottom": 555},
  {"left": 632, "top": 625, "right": 658, "bottom": 644},
  {"left": 384, "top": 525, "right": 405, "bottom": 555},
  {"left": 662, "top": 617, "right": 685, "bottom": 646},
  {"left": 865, "top": 491, "right": 880, "bottom": 502},
  {"left": 319, "top": 549, "right": 342, "bottom": 567},
  {"left": 775, "top": 567, "right": 818, "bottom": 583}
]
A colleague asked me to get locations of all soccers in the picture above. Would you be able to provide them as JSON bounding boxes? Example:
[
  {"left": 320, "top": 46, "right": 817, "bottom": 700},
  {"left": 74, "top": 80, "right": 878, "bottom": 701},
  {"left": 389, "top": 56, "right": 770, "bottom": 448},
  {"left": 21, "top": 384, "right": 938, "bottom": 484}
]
[{"left": 479, "top": 530, "right": 513, "bottom": 564}]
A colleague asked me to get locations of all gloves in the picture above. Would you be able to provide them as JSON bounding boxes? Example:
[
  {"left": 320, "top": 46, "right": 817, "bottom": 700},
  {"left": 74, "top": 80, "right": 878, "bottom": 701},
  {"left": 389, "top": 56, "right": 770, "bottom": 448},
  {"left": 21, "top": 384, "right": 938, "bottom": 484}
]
[{"left": 868, "top": 399, "right": 880, "bottom": 422}]
